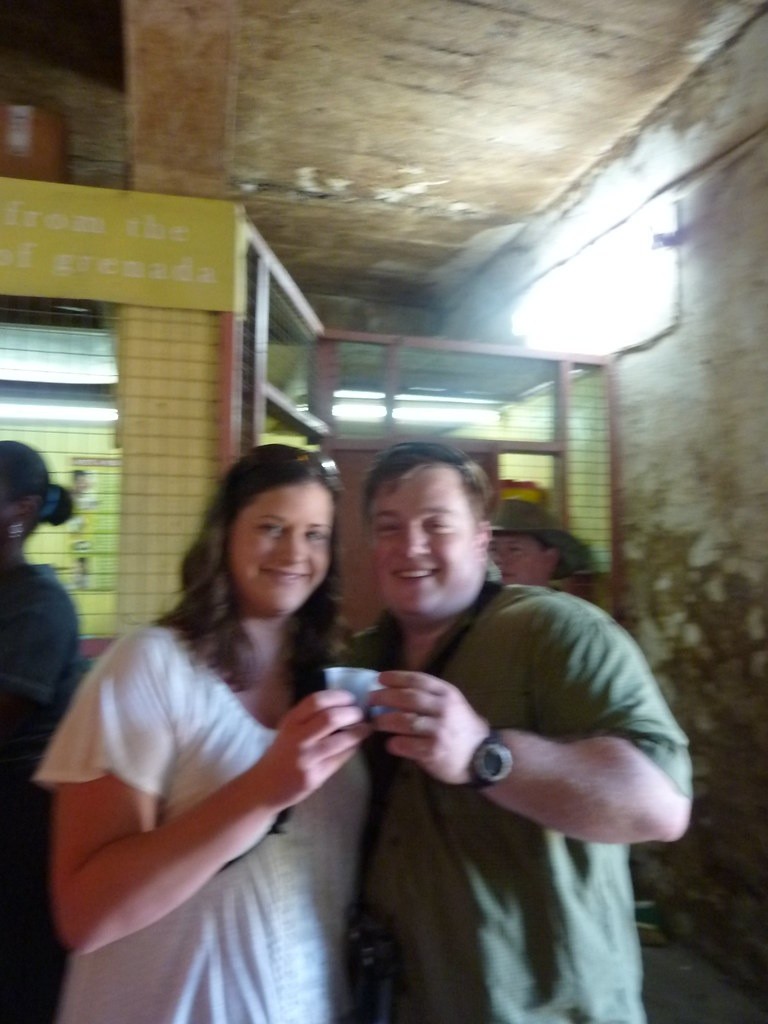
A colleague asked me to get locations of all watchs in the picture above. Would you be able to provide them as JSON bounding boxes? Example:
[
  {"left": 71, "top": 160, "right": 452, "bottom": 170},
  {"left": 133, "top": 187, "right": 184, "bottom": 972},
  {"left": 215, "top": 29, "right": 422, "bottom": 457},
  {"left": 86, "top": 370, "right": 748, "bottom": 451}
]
[{"left": 468, "top": 726, "right": 514, "bottom": 789}]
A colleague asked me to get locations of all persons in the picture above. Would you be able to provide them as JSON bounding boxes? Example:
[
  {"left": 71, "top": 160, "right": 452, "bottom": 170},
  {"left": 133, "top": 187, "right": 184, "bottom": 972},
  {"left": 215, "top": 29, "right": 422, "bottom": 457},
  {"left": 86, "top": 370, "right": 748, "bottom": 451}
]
[
  {"left": 30, "top": 443, "right": 369, "bottom": 1024},
  {"left": 487, "top": 496, "right": 596, "bottom": 597},
  {"left": 316, "top": 440, "right": 693, "bottom": 1023},
  {"left": 1, "top": 440, "right": 90, "bottom": 1024}
]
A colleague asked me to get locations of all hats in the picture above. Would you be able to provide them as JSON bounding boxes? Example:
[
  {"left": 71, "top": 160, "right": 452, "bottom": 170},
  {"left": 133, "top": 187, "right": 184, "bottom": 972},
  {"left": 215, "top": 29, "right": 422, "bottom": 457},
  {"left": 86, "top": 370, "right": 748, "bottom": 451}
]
[{"left": 492, "top": 498, "right": 592, "bottom": 581}]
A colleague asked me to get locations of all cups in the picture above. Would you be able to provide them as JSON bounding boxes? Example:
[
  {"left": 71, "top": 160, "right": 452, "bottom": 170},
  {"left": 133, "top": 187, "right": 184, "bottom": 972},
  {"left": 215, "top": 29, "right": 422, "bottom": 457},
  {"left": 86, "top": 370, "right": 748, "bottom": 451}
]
[{"left": 324, "top": 666, "right": 379, "bottom": 730}]
